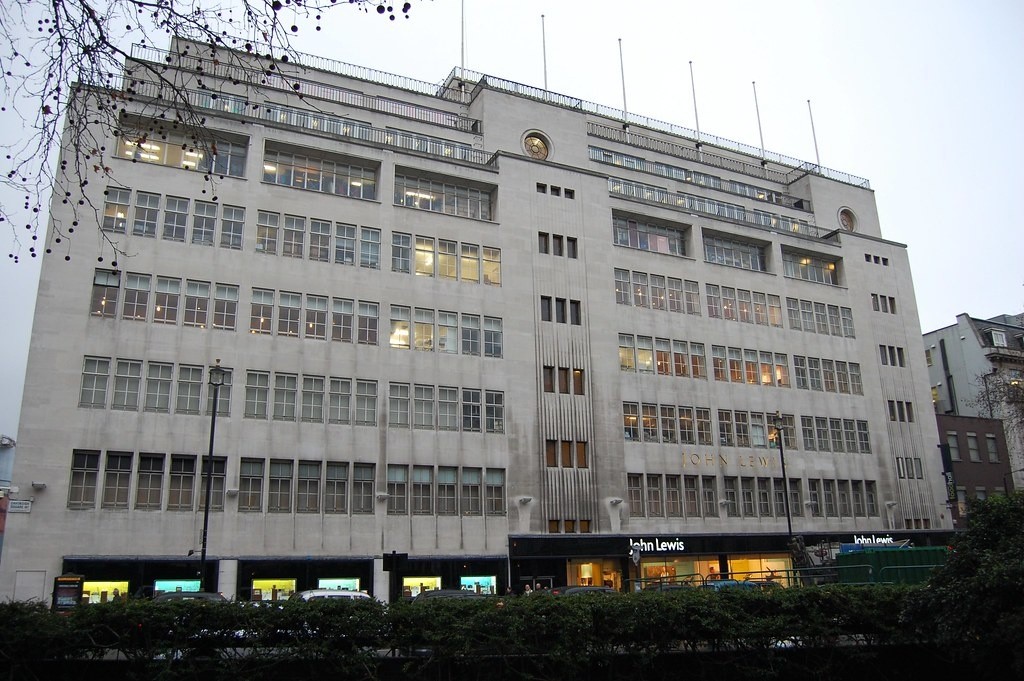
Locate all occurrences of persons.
[507,587,514,596]
[710,567,718,579]
[536,583,541,591]
[524,584,533,596]
[112,588,121,601]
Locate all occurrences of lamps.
[31,481,46,489]
[610,499,623,505]
[520,497,532,503]
[377,495,389,500]
[227,490,240,496]
[805,500,817,507]
[719,499,731,506]
[886,502,899,508]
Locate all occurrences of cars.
[150,567,762,604]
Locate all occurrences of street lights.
[772,411,800,587]
[199,357,225,592]
